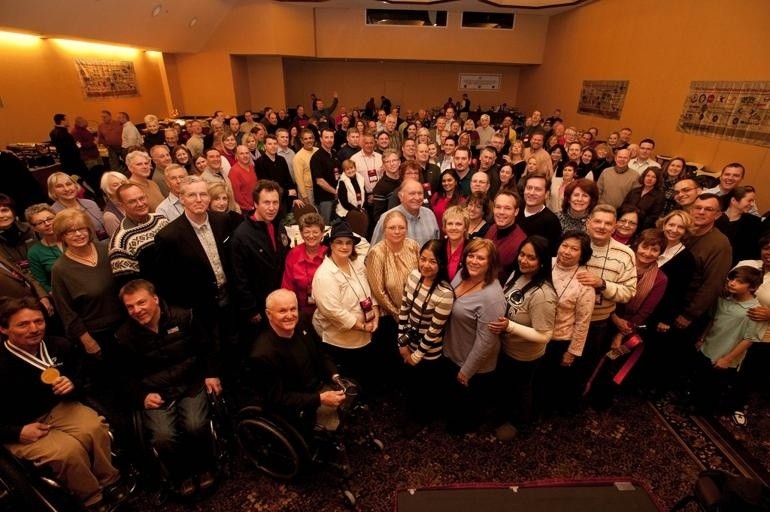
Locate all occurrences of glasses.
[30,215,54,228]
[62,225,88,235]
[673,186,697,196]
[618,219,638,226]
[690,204,719,211]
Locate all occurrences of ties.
[201,226,225,284]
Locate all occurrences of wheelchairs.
[131,387,228,497]
[230,376,385,509]
[0,396,137,512]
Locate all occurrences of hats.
[321,221,361,246]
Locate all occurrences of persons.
[1,94,769,512]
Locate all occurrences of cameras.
[397,327,423,348]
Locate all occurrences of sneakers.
[107,481,129,504]
[732,410,747,426]
[199,470,215,488]
[496,423,516,441]
[180,476,195,496]
[92,501,111,512]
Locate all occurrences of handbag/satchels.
[345,209,369,237]
